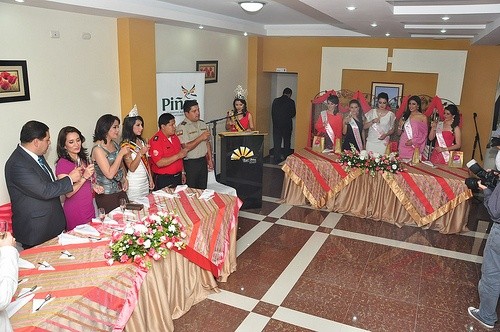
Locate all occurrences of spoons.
[61,252,73,257]
[38,263,50,268]
[18,285,37,298]
[36,294,51,311]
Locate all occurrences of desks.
[6,186,239,332]
[278,147,475,235]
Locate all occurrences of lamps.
[240,2,265,13]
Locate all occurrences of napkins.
[7,184,216,322]
[421,161,433,167]
[322,149,334,152]
[402,158,412,163]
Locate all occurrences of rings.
[14,238,15,240]
[409,144,410,145]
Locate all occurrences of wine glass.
[322,110,439,131]
[206,126,212,142]
[87,156,95,180]
[120,198,127,221]
[123,139,130,159]
[183,142,188,159]
[98,208,105,234]
[144,138,150,157]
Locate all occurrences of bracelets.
[398,127,401,130]
[82,176,88,180]
[345,122,347,125]
[138,152,142,157]
[181,172,186,175]
[386,133,389,136]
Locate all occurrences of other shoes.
[468,306,494,328]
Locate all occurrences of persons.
[90,114,131,215]
[148,113,188,191]
[468,168,500,328]
[120,104,155,201]
[55,126,104,232]
[495,135,500,171]
[429,104,461,166]
[4,120,83,251]
[226,85,255,131]
[271,88,296,163]
[342,99,366,153]
[0,232,18,332]
[363,92,396,153]
[175,100,213,190]
[315,95,342,150]
[396,95,428,159]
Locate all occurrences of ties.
[39,158,53,182]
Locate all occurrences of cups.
[74,158,82,167]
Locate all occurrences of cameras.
[465,159,498,193]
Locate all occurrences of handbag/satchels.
[126,203,144,210]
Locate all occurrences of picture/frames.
[0,59,30,103]
[196,59,219,83]
[370,82,403,110]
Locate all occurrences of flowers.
[102,211,188,267]
[341,150,399,171]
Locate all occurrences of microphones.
[241,109,246,115]
[473,113,477,119]
